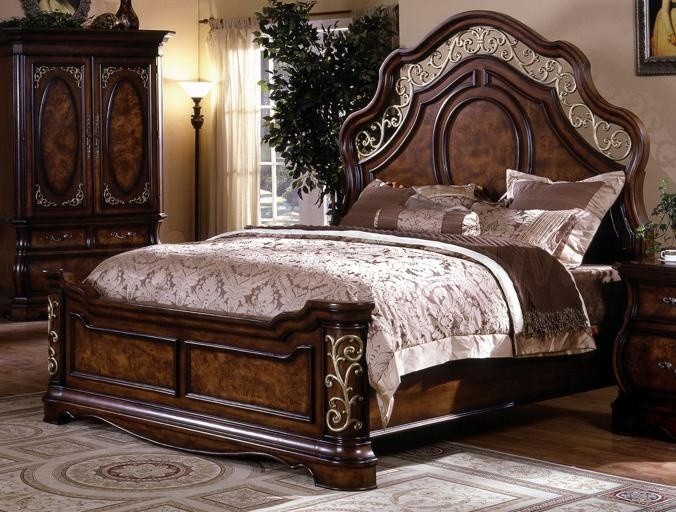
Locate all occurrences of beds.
[41,10,654,492]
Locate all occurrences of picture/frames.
[635,0,676,78]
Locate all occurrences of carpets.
[0,389,676,512]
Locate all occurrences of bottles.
[116,0,140,30]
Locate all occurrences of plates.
[658,258,676,265]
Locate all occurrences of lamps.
[179,81,214,241]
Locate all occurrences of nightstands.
[607,254,676,444]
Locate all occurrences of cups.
[630,233,646,264]
[661,250,676,260]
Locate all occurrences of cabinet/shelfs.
[0,0,176,319]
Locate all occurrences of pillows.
[330,168,625,268]
